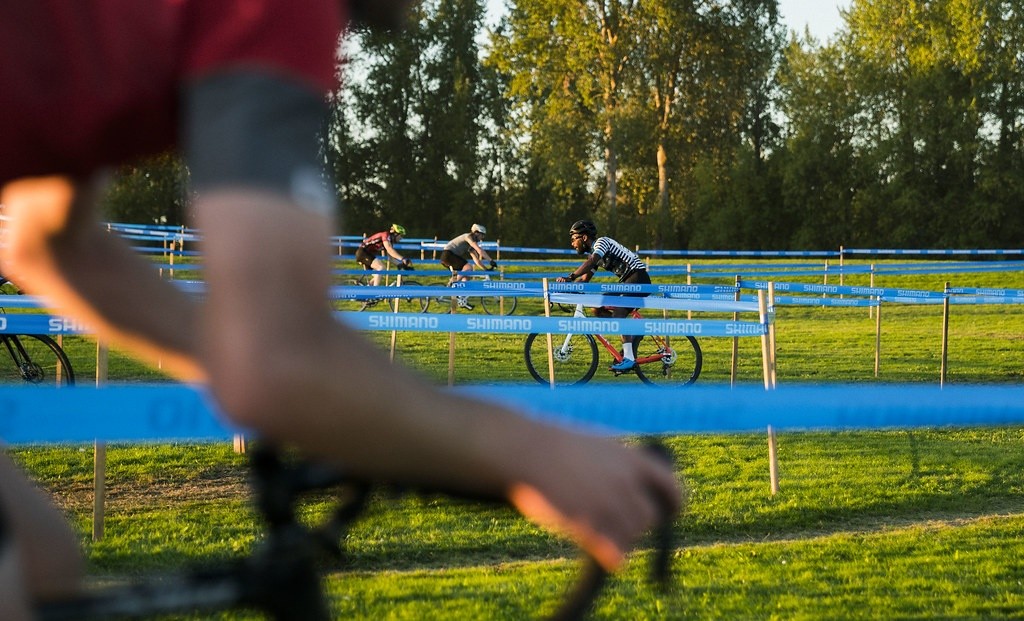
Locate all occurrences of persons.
[350,224,412,301]
[0,0,680,621]
[441,223,497,311]
[555,219,652,371]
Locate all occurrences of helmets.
[390,224,405,236]
[569,220,597,237]
[471,223,486,234]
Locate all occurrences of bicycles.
[0,278,75,387]
[524,278,704,391]
[420,264,518,318]
[328,260,430,314]
[0,436,679,621]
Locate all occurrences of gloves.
[490,261,497,267]
[402,257,409,265]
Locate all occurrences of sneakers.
[611,357,637,370]
